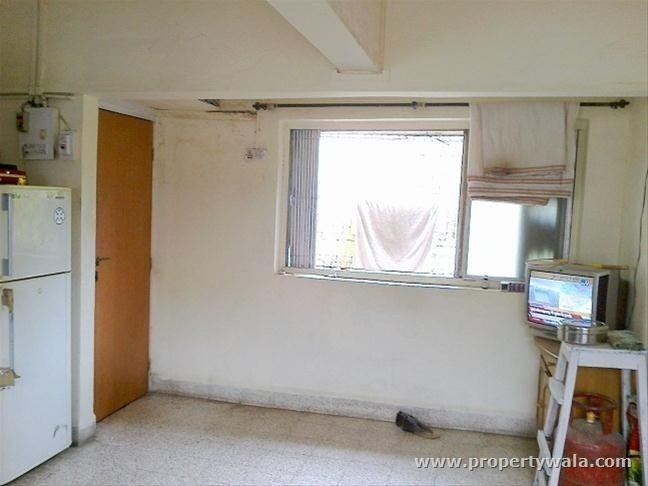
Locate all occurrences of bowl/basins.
[556,317,610,345]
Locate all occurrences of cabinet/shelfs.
[532,337,636,447]
[531,341,648,486]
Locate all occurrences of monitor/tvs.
[523,257,634,344]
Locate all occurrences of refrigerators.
[0,184,73,485]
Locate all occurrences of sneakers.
[397,411,440,439]
